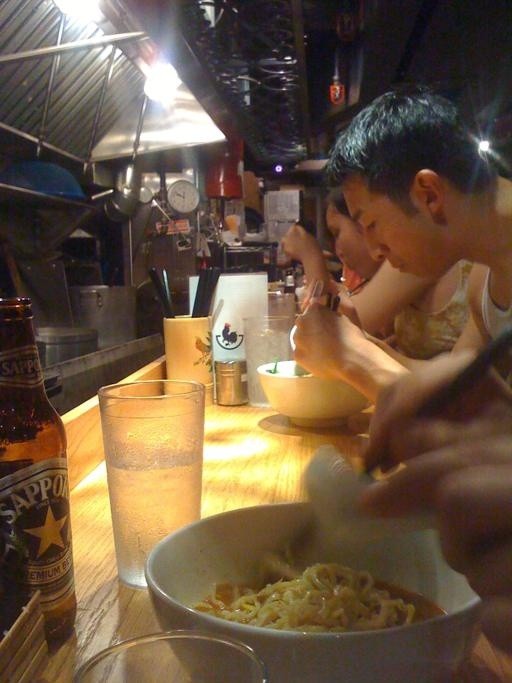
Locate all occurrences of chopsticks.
[146,261,220,318]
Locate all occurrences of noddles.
[189,563,417,634]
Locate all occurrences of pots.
[71,284,139,348]
[33,326,99,368]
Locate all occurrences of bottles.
[1,294,76,650]
[284,268,302,311]
[215,359,249,407]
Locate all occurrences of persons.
[281,183,476,366]
[360,344,511,658]
[286,81,511,405]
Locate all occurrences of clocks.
[167,180,200,213]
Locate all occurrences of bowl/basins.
[255,358,375,427]
[142,496,491,682]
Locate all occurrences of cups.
[242,312,292,407]
[271,293,299,324]
[69,627,275,681]
[96,377,208,593]
[162,313,218,405]
